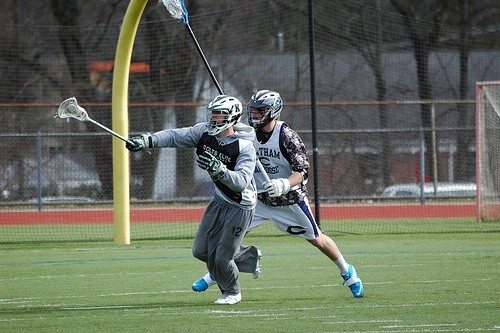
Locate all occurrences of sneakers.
[214,292,242,305]
[192,273,218,292]
[251,245,262,279]
[340,264,364,298]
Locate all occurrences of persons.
[191,89,364,298]
[126,95,263,304]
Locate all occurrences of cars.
[0,147,105,203]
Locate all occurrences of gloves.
[196,151,227,182]
[125,133,155,152]
[261,178,290,197]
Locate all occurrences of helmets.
[204,95,243,136]
[247,89,283,128]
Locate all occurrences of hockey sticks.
[160,0,226,95]
[55,96,139,146]
